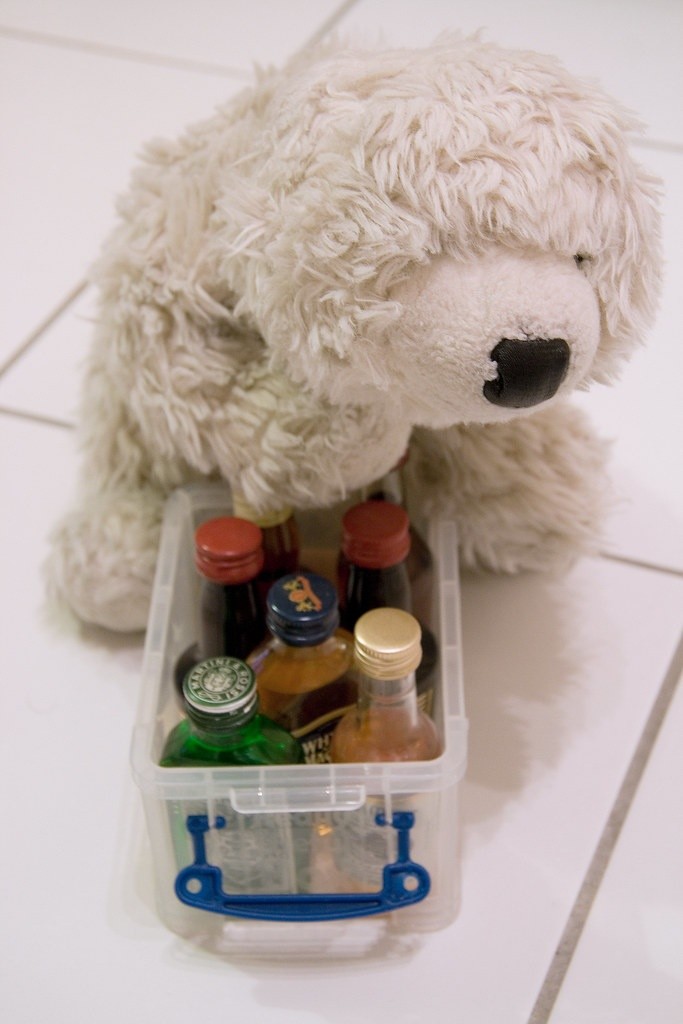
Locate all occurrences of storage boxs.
[128,479,469,956]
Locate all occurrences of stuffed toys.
[41,28,662,640]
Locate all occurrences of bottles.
[327,605,445,920]
[334,445,439,649]
[335,500,437,737]
[238,569,360,837]
[159,655,311,912]
[227,481,312,590]
[176,517,282,705]
